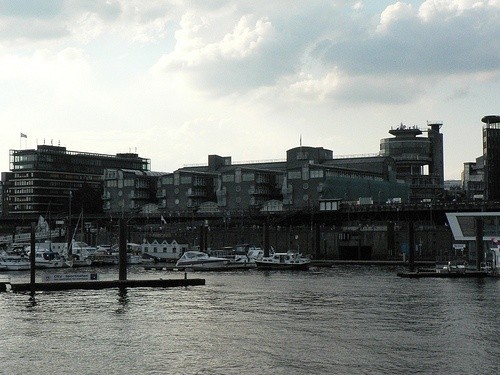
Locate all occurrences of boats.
[256,252,311,270]
[0,216,274,272]
